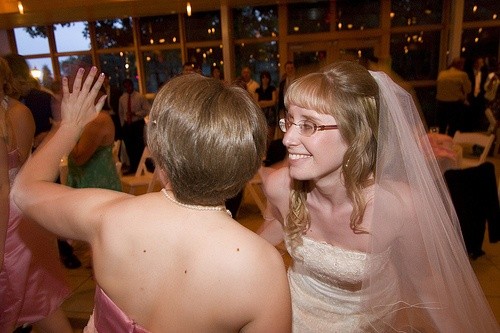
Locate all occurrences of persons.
[0,52,500,269]
[1,60,70,333]
[260,59,500,333]
[13,63,293,333]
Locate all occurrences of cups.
[430,127,439,136]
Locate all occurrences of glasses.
[278,117,339,137]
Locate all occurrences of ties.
[128,94,132,127]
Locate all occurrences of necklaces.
[161,188,233,218]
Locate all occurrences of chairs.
[58,130,496,258]
